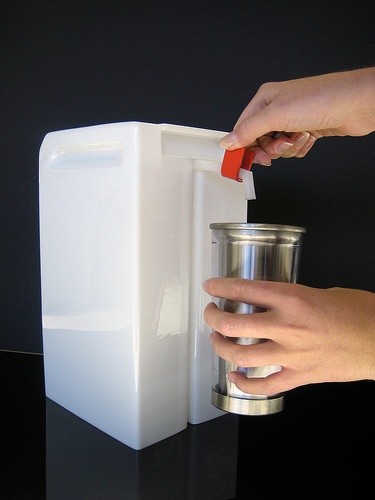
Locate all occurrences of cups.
[208,223,307,420]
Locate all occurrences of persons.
[204,65,375,396]
